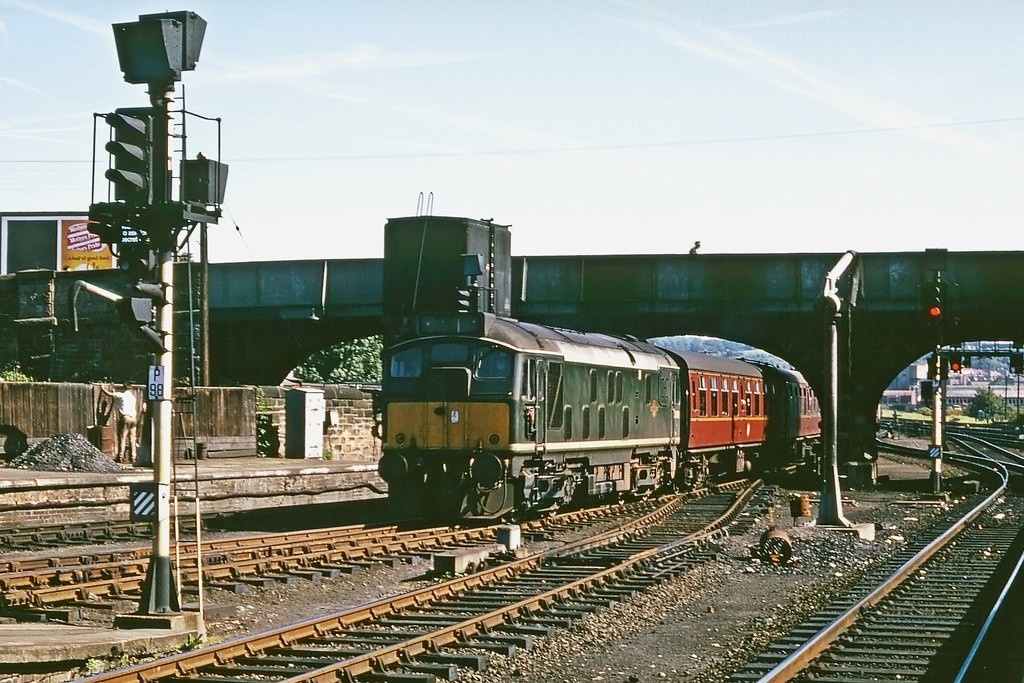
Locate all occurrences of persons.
[689,241,701,254]
[101,380,137,463]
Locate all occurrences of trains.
[371,312,826,528]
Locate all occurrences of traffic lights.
[950,352,962,372]
[454,284,480,316]
[114,295,154,331]
[104,107,154,206]
[928,280,944,324]
[964,354,971,368]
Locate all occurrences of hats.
[695,241,700,245]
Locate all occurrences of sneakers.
[130,458,137,464]
[113,457,122,463]
